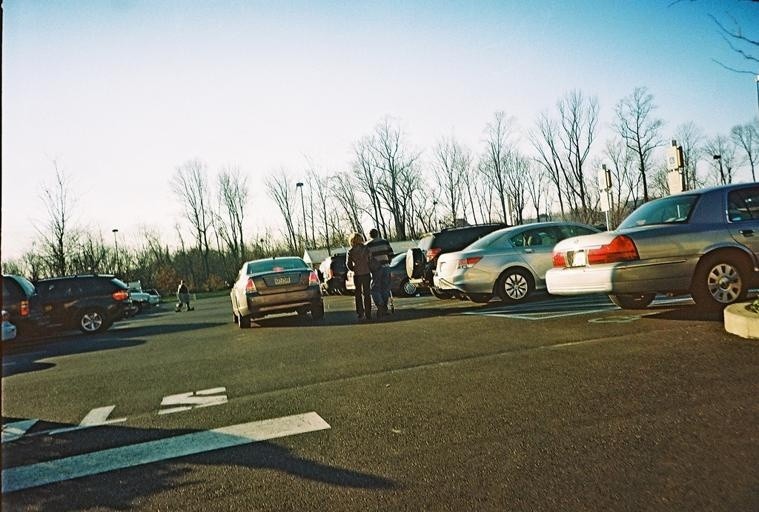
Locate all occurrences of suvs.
[315,253,347,295]
[406,223,513,301]
[33,274,133,335]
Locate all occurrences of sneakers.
[357,307,391,321]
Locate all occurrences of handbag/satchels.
[367,249,381,272]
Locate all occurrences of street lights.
[297,183,307,245]
[113,229,120,274]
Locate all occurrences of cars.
[0,274,48,355]
[545,182,759,312]
[433,220,602,306]
[128,285,161,315]
[229,256,324,328]
[371,251,419,298]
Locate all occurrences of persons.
[363,228,395,317]
[175,280,194,313]
[345,232,372,320]
